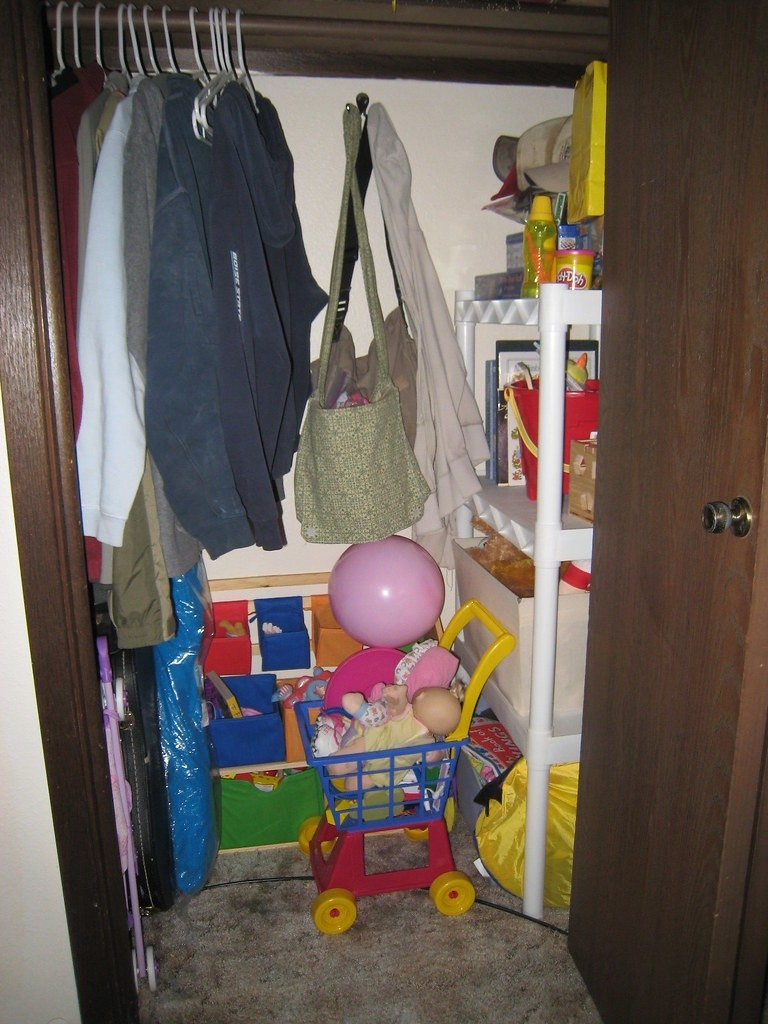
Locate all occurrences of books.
[206,670,243,718]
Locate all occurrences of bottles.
[520,195,555,299]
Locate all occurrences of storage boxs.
[204,600,252,676]
[450,537,588,717]
[311,591,363,667]
[569,439,596,521]
[214,767,325,851]
[255,595,311,671]
[474,272,523,300]
[506,225,576,272]
[566,61,607,225]
[457,749,490,833]
[278,678,323,763]
[208,674,286,769]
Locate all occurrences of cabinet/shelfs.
[453,283,603,919]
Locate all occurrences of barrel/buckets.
[505,374,602,501]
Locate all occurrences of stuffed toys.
[312,638,460,766]
[328,678,464,791]
[465,513,573,600]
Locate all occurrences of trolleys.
[299,599,516,934]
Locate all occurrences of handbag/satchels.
[294,378,432,545]
[308,306,419,446]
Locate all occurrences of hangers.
[51,0,257,144]
